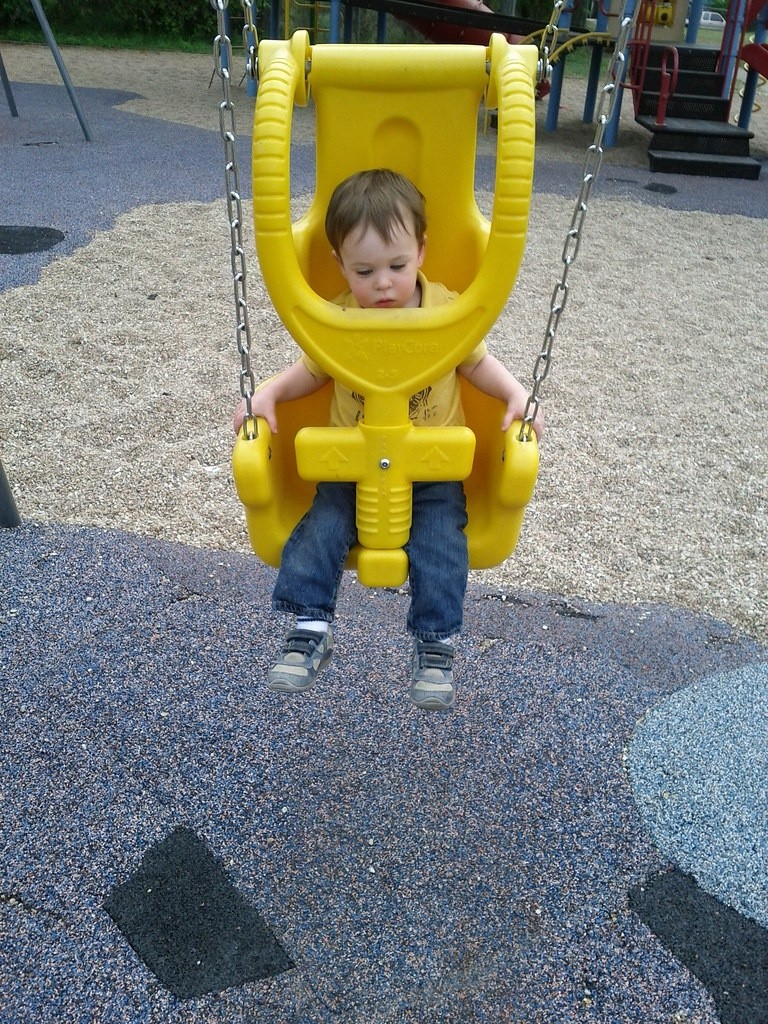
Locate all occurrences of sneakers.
[267,626,333,692]
[409,639,455,710]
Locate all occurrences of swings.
[207,0,639,591]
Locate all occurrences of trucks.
[685,11,726,29]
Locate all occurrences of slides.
[740,42,768,81]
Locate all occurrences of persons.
[231,168,545,707]
